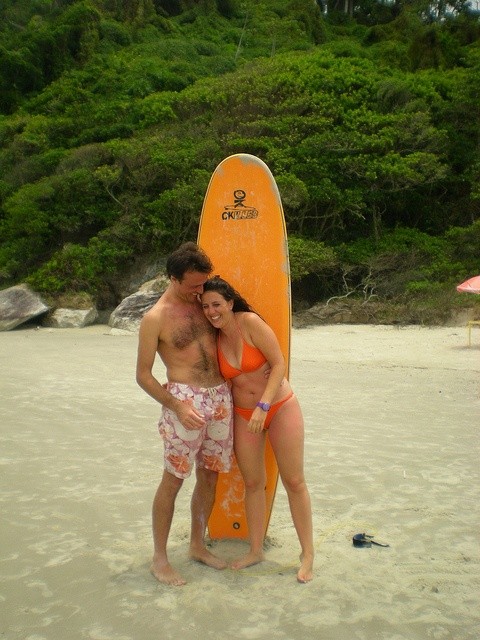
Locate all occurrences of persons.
[136,241,234,586]
[199,274,315,584]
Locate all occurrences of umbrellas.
[456,276,480,295]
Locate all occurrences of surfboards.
[196,153,291,540]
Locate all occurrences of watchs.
[256,402,271,411]
